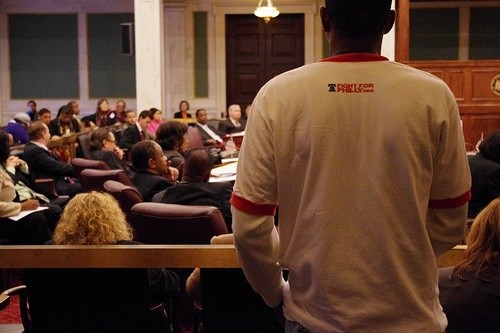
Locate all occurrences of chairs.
[35,158,229,333]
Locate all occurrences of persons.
[6,101,86,159]
[3,156,71,222]
[230,0,471,332]
[26,190,180,333]
[468,132,500,219]
[88,128,135,182]
[153,148,233,236]
[0,129,51,246]
[437,200,500,333]
[23,123,84,195]
[195,109,229,147]
[157,121,190,182]
[219,104,247,134]
[87,98,164,149]
[131,140,177,201]
[174,100,192,119]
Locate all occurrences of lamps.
[254,0,280,24]
